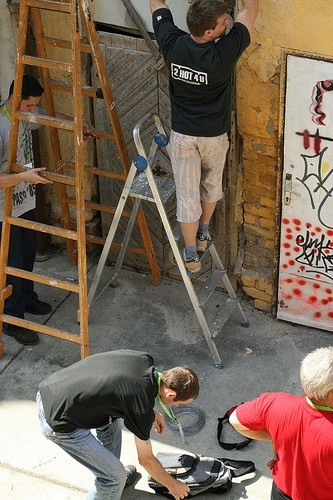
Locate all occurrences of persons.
[229,346,333,500]
[35,348,200,500]
[0,76,53,347]
[149,0,261,272]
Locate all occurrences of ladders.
[77,112,250,369]
[0,0,163,366]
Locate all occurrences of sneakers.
[2,322,39,346]
[169,247,201,272]
[24,299,51,315]
[196,238,208,251]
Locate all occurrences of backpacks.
[148,451,256,498]
